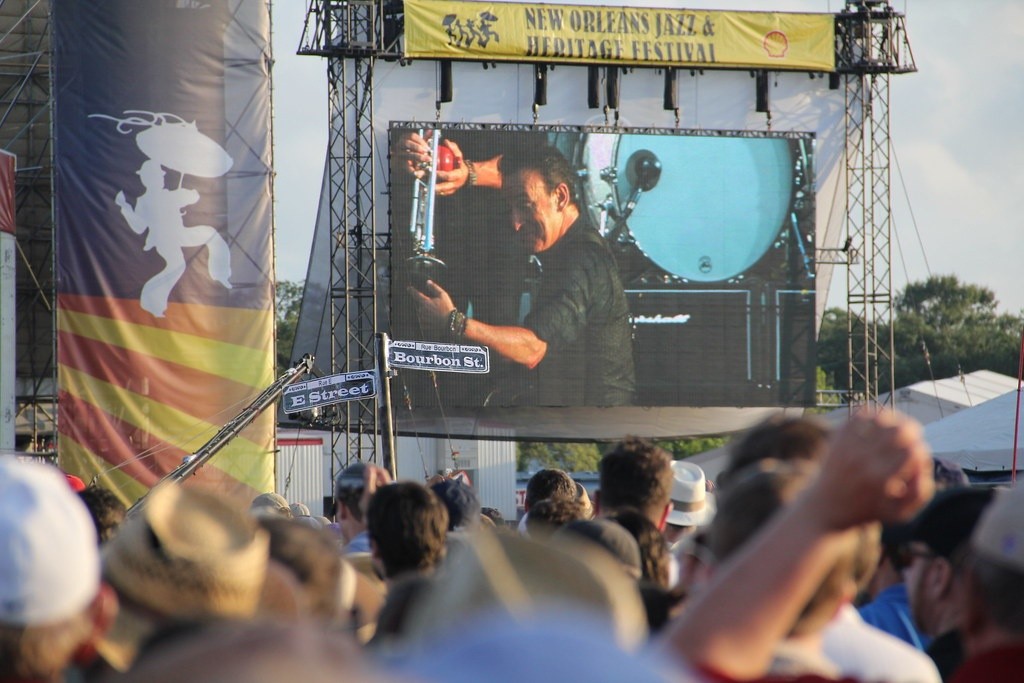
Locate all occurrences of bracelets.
[463,158,478,191]
[449,309,466,338]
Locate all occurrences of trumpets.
[403,129,451,289]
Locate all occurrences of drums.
[571,133,793,285]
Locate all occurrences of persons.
[395,127,547,328]
[406,142,642,407]
[0,399,1024,683]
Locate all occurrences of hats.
[103,479,271,630]
[397,517,655,656]
[665,459,718,528]
[0,452,101,628]
[970,479,1024,569]
